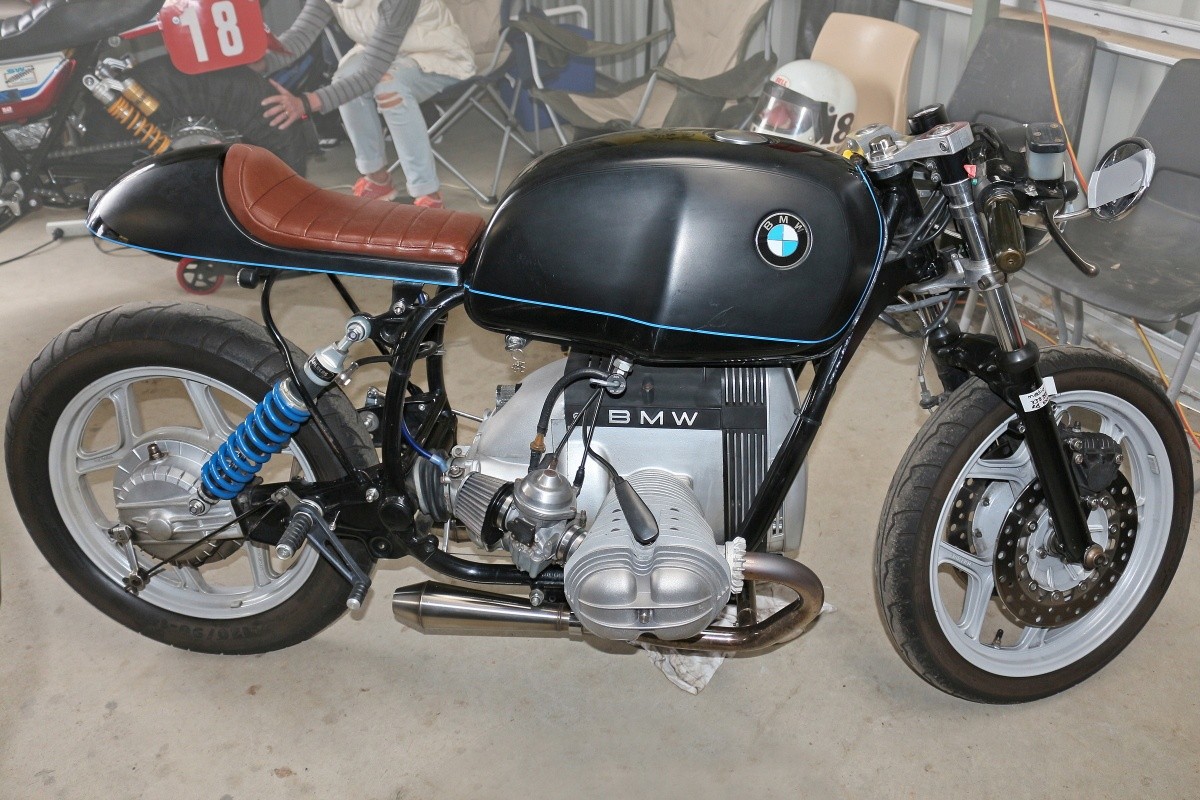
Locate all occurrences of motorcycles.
[0,0,308,233]
[6,105,1194,704]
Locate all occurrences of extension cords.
[46,219,92,238]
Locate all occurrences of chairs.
[876,16,1099,347]
[971,59,1200,495]
[325,0,587,205]
[796,0,901,73]
[811,12,921,162]
[508,0,778,142]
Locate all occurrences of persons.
[249,0,478,208]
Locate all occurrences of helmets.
[749,58,855,154]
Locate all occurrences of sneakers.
[413,193,443,208]
[350,174,397,201]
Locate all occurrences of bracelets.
[296,92,312,114]
